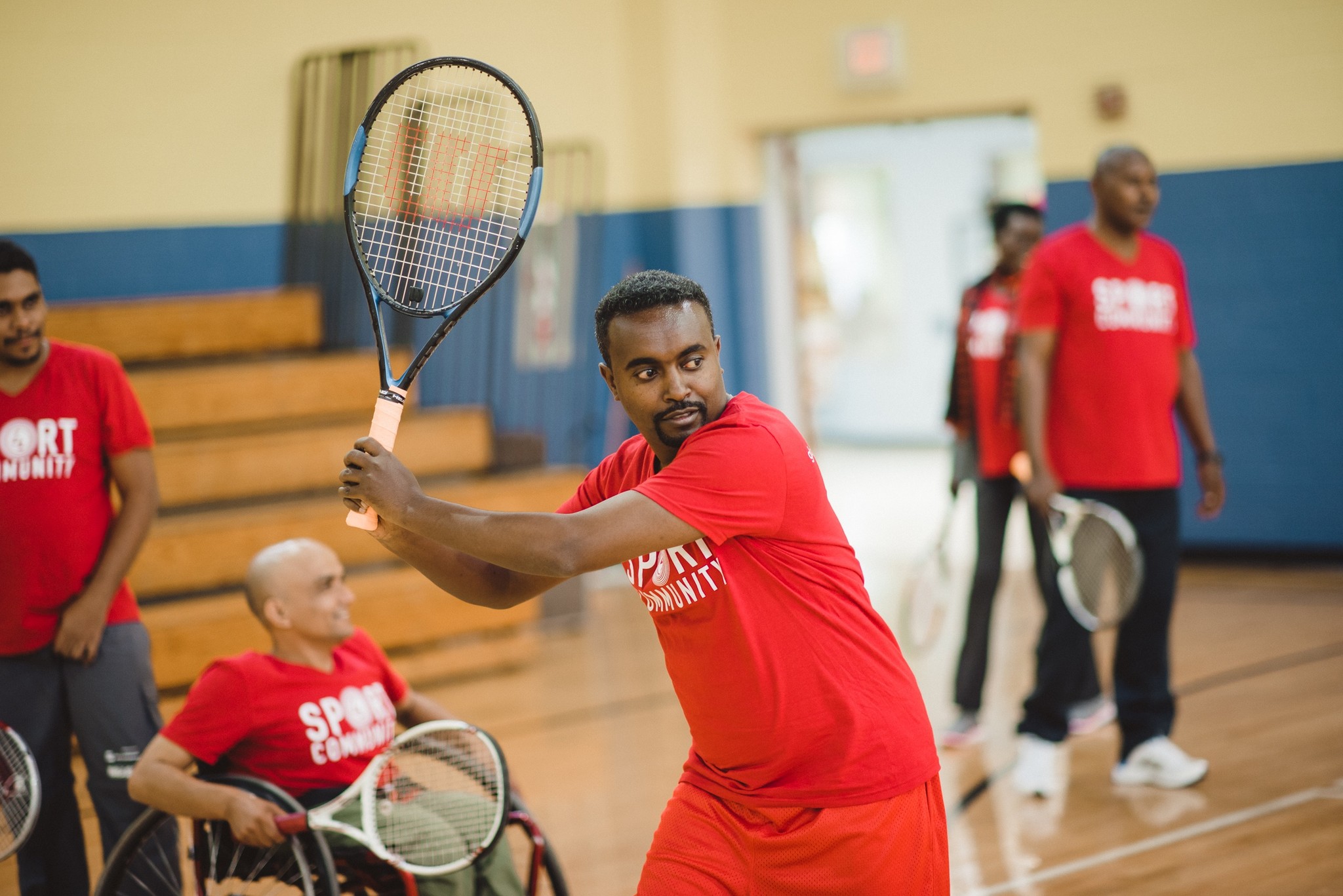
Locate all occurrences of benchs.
[34,282,589,715]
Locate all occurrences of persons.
[940,200,1117,755]
[0,241,182,896]
[1003,143,1228,798]
[129,538,528,896]
[340,267,951,896]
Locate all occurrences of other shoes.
[1012,733,1065,797]
[1068,690,1118,737]
[938,702,979,750]
[1110,733,1211,788]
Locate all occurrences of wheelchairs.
[93,716,567,896]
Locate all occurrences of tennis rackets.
[1009,447,1143,631]
[272,717,507,878]
[345,54,544,531]
[901,474,965,658]
[0,721,40,860]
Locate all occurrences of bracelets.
[1195,448,1224,467]
